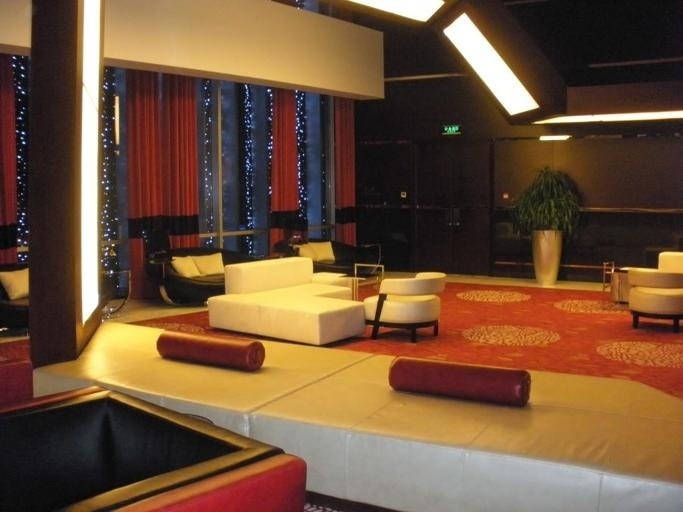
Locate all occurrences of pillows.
[0,267,28,299]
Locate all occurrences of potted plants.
[506,165,583,289]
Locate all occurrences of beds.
[152,236,379,300]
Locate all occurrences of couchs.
[207,255,365,344]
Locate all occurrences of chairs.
[364,269,446,341]
[627,251,681,329]
